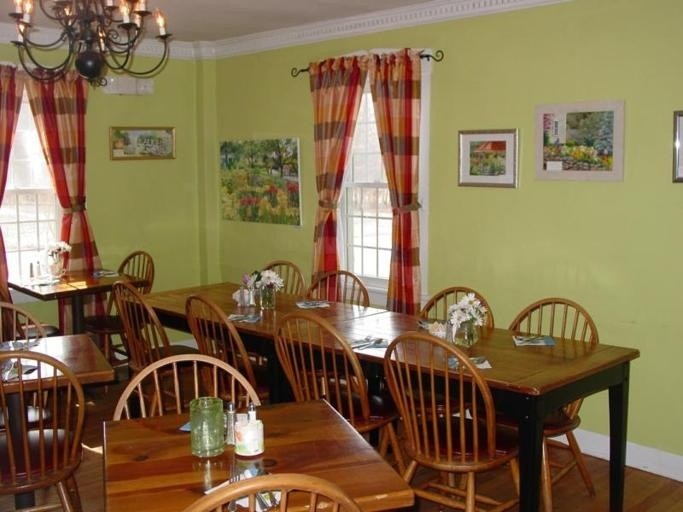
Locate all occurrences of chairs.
[479,297,598,512]
[0,350,84,512]
[273,311,406,477]
[8,291,60,338]
[82,249,155,365]
[0,301,46,429]
[421,284,495,329]
[185,295,271,410]
[112,280,208,417]
[114,354,262,421]
[384,331,520,512]
[308,271,370,308]
[262,258,305,299]
[184,472,365,512]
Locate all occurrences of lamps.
[0,0,173,88]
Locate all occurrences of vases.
[51,259,66,280]
[456,322,479,349]
[258,288,276,310]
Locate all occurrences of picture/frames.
[458,126,520,188]
[217,137,306,226]
[533,100,627,178]
[109,125,175,160]
[673,109,682,184]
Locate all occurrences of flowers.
[242,268,285,311]
[47,240,73,280]
[444,291,487,347]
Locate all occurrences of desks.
[0,335,114,433]
[101,398,414,512]
[124,281,640,511]
[9,268,152,337]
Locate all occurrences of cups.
[188,396,231,458]
[238,287,262,324]
